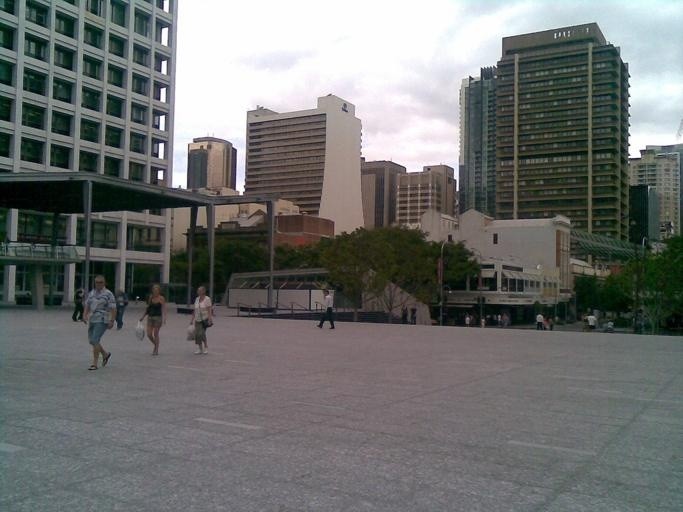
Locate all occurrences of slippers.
[103,351,111,366]
[88,365,97,370]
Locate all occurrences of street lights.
[641,237,650,334]
[470,248,482,328]
[438,240,454,326]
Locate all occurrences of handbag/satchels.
[202,319,213,328]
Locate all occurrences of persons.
[139,284,166,355]
[316,290,335,329]
[583,313,597,330]
[537,314,554,329]
[115,288,128,330]
[604,319,614,333]
[72,288,84,322]
[464,311,510,328]
[190,286,212,354]
[83,275,117,369]
